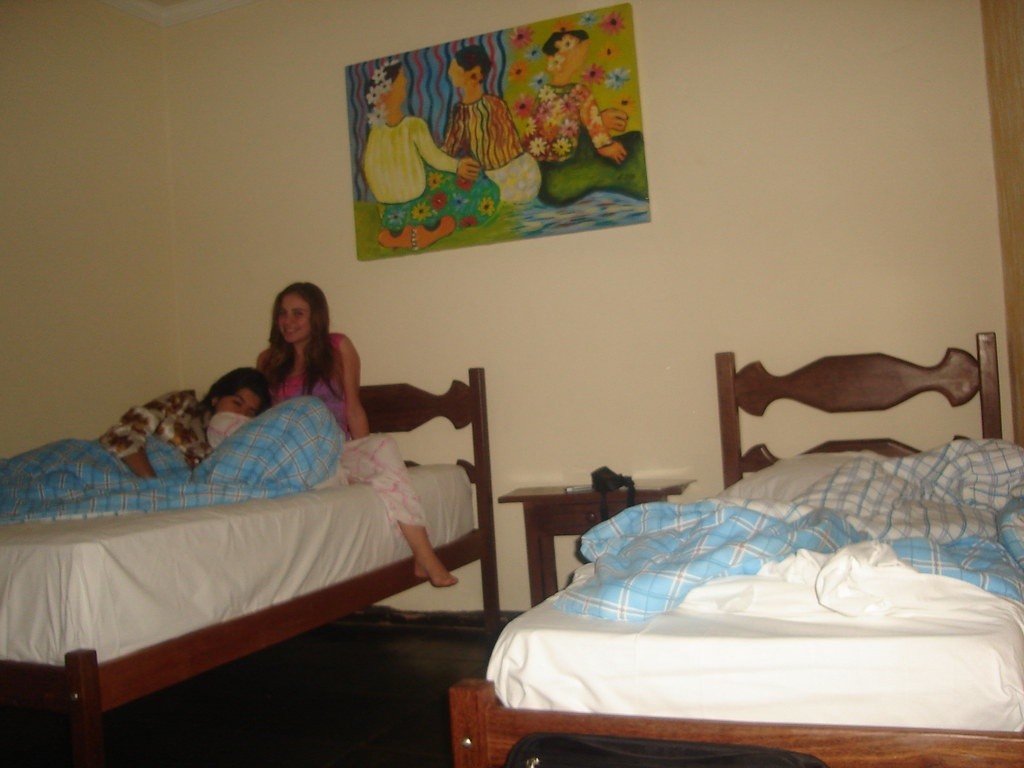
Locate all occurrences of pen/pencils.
[564,485,592,491]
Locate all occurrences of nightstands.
[498,479,696,610]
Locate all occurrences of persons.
[99,368,272,476]
[254,282,460,588]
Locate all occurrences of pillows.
[711,450,862,501]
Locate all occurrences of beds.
[0,366,497,768]
[447,332,1024,768]
